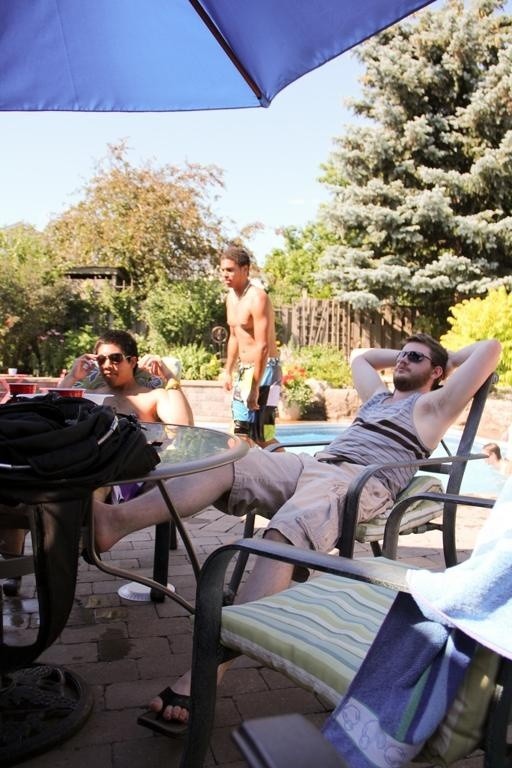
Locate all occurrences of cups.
[9,383,38,404]
[48,389,87,398]
[9,368,18,377]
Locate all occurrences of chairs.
[223,372,500,607]
[0,354,182,604]
[178,491,511,768]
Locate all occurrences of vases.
[279,396,301,421]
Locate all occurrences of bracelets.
[161,377,180,391]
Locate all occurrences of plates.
[118,581,176,602]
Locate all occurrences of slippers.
[137,685,191,739]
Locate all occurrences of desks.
[1,421,251,767]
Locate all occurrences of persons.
[217,244,287,454]
[56,329,196,505]
[480,442,512,476]
[88,330,503,739]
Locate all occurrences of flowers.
[276,367,311,407]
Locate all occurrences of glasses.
[96,350,128,365]
[397,350,436,369]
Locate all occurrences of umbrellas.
[2,0,446,116]
